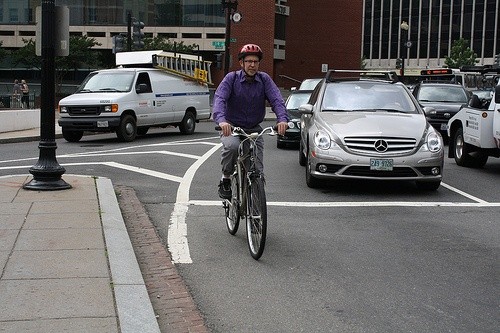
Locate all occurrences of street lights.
[399,19,409,83]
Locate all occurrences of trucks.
[449,79,500,167]
[58,67,211,143]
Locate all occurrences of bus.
[421,68,500,92]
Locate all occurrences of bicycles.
[214,124,290,259]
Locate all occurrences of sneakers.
[250,215,261,234]
[218,178,232,198]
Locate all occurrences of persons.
[12,80,22,108]
[20,80,30,109]
[212,43,288,234]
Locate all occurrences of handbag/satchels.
[23,84,28,93]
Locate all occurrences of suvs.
[298,69,445,192]
[412,75,469,143]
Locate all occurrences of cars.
[270,92,314,149]
[299,79,323,95]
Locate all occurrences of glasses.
[244,60,259,63]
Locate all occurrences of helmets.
[238,44,263,59]
